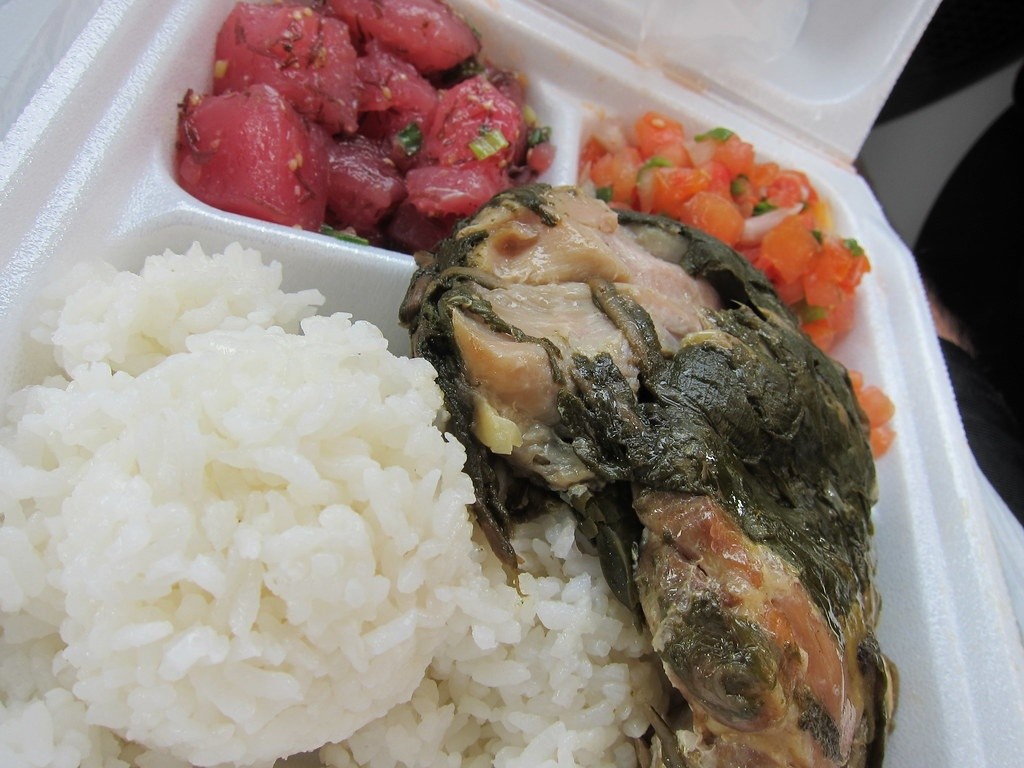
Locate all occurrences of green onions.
[395,102,865,322]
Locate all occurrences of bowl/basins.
[0,0,1024,768]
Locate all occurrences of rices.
[0,239,670,768]
